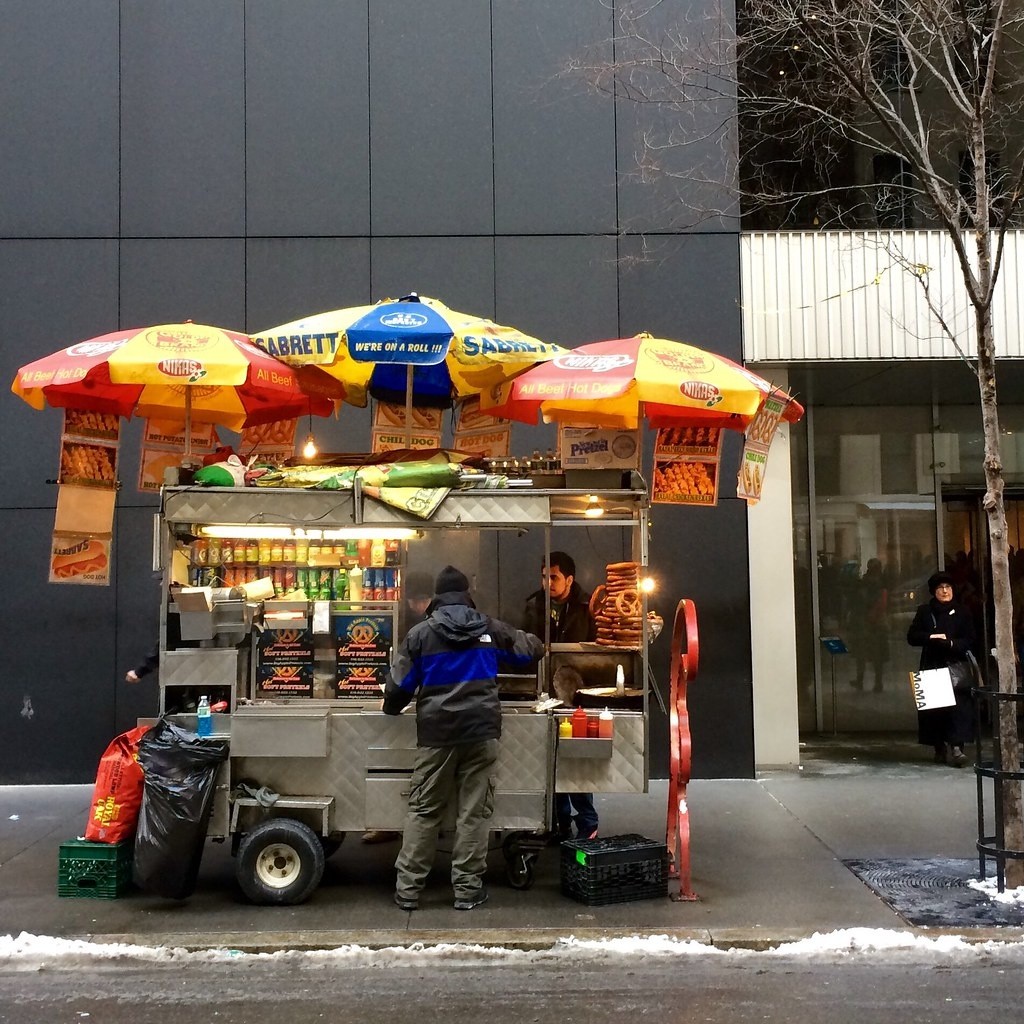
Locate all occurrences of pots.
[575,682,652,709]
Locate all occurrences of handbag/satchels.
[908,651,986,710]
[869,589,890,632]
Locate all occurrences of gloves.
[930,638,952,651]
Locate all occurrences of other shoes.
[850,679,883,693]
[362,831,396,842]
[933,746,967,767]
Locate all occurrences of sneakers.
[394,891,419,910]
[455,891,489,909]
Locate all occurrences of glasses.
[936,586,951,590]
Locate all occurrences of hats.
[435,566,468,593]
[405,571,434,599]
[929,573,956,595]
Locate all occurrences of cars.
[891,565,936,612]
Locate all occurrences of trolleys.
[157,481,663,908]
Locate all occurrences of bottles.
[197,696,212,737]
[588,717,599,738]
[559,717,572,737]
[189,539,398,567]
[570,705,587,738]
[599,706,613,738]
[349,563,362,610]
[334,569,349,610]
[488,447,563,474]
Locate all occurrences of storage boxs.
[56,834,133,902]
[561,831,673,907]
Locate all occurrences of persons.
[816,548,1024,695]
[125,572,197,716]
[907,571,970,765]
[381,565,545,909]
[402,571,435,639]
[519,550,598,843]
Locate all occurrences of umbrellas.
[12,320,345,460]
[250,292,569,447]
[481,332,806,480]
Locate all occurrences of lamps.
[585,496,604,519]
[190,522,425,542]
